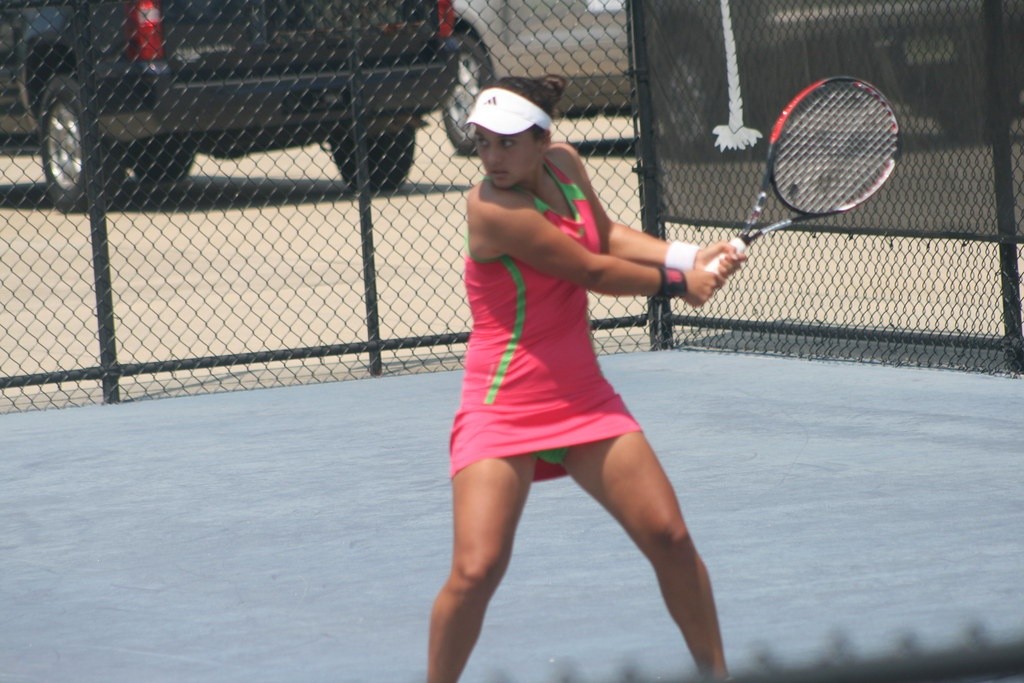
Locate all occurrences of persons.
[425,72,747,682]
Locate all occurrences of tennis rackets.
[701,74,902,275]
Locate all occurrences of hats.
[464,86,552,135]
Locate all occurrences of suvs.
[0,1,462,213]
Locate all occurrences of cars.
[442,2,1023,159]
